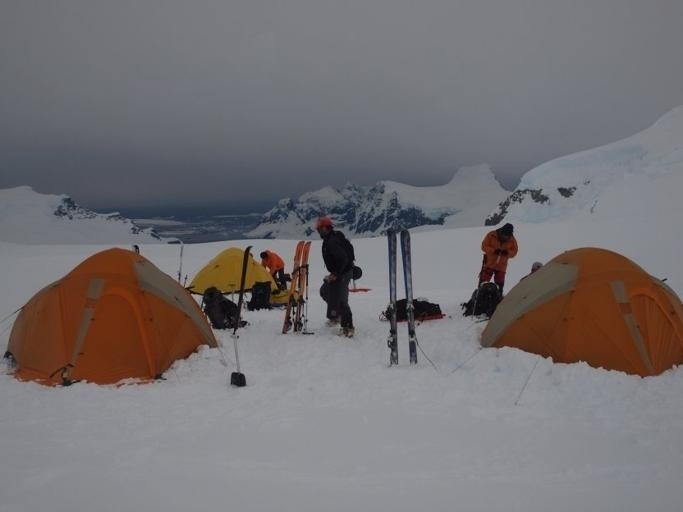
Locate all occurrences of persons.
[316,218,354,338]
[532,262,542,273]
[260,250,287,290]
[478,222,518,296]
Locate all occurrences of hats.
[532,262,541,269]
[260,252,266,258]
[503,224,513,233]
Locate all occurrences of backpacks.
[201,286,246,329]
[247,281,271,311]
[461,283,501,317]
[379,299,440,322]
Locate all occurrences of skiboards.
[233,246,252,333]
[178,240,184,284]
[381,228,417,366]
[282,241,311,334]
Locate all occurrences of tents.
[188,248,278,295]
[481,247,683,378]
[4,248,218,386]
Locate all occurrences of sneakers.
[339,327,354,338]
[325,318,341,327]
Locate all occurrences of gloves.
[501,250,507,256]
[495,248,500,255]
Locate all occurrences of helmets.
[318,217,333,226]
